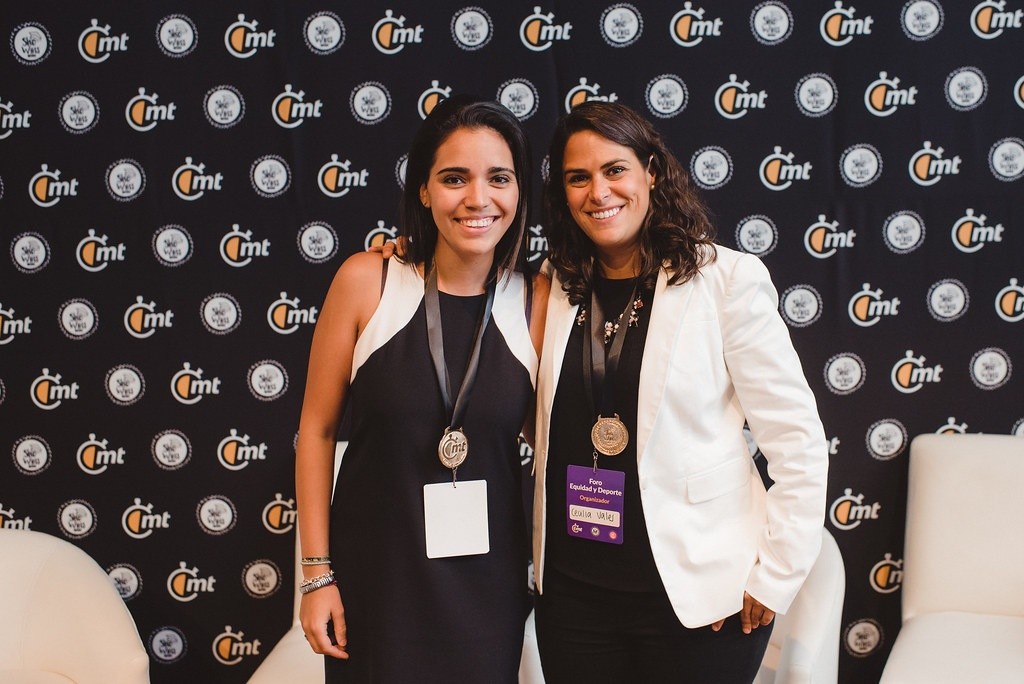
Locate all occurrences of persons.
[294,90,552,684]
[365,100,829,684]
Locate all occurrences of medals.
[438,425,468,469]
[591,412,629,456]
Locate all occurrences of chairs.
[246,442,348,684]
[0,527,151,684]
[518,527,846,684]
[879,434,1024,684]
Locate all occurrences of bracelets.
[298,570,335,588]
[299,575,335,594]
[301,556,331,565]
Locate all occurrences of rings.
[304,634,308,641]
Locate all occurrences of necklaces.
[576,292,643,345]
